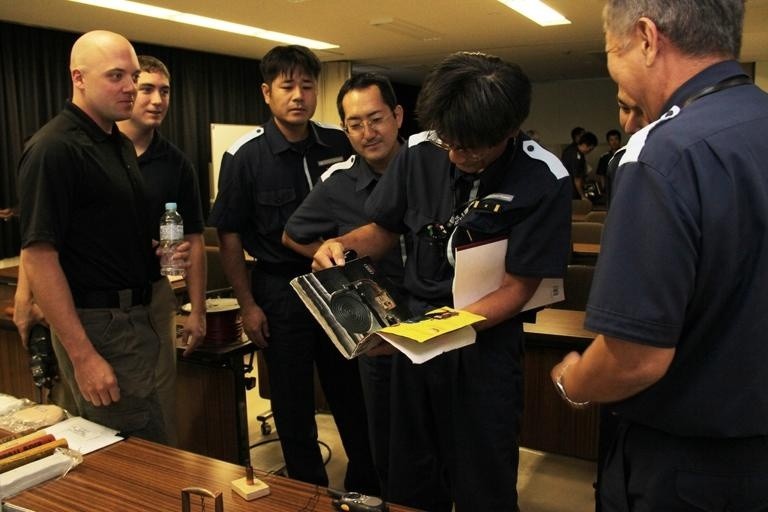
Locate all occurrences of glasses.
[343,114,391,137]
[427,128,491,162]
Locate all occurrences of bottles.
[157,200,191,281]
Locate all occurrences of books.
[289,254,487,365]
[452,235,566,334]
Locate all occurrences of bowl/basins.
[182,297,251,347]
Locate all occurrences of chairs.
[570,199,607,244]
[551,265,595,311]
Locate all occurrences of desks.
[520,306,600,463]
[0,431,416,510]
[0,256,250,466]
[571,242,601,265]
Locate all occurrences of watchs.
[555,366,594,410]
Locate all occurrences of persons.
[13,54,209,449]
[311,51,572,512]
[527,127,622,211]
[550,0,768,512]
[17,30,192,448]
[593,82,652,512]
[211,46,381,498]
[282,74,410,498]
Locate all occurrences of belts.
[73,290,153,310]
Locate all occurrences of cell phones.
[328,489,387,512]
